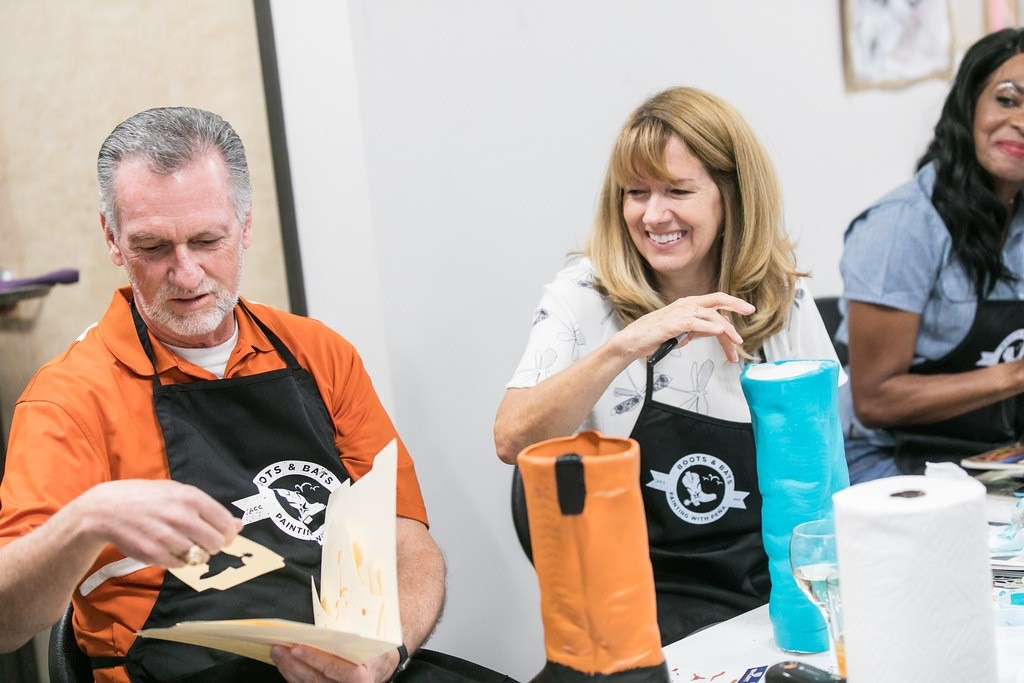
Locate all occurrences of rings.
[694,305,699,318]
[183,544,203,566]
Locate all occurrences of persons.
[493,87,909,648]
[835,30,1024,487]
[0,106,525,683]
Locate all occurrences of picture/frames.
[983,1,1020,36]
[840,0,959,93]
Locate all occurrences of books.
[960,442,1024,469]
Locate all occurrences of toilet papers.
[831,462,998,683]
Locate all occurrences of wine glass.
[790,520,847,680]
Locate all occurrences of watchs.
[389,644,410,683]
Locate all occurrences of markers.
[646,332,689,368]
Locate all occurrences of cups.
[986,481,1024,559]
[822,565,846,679]
